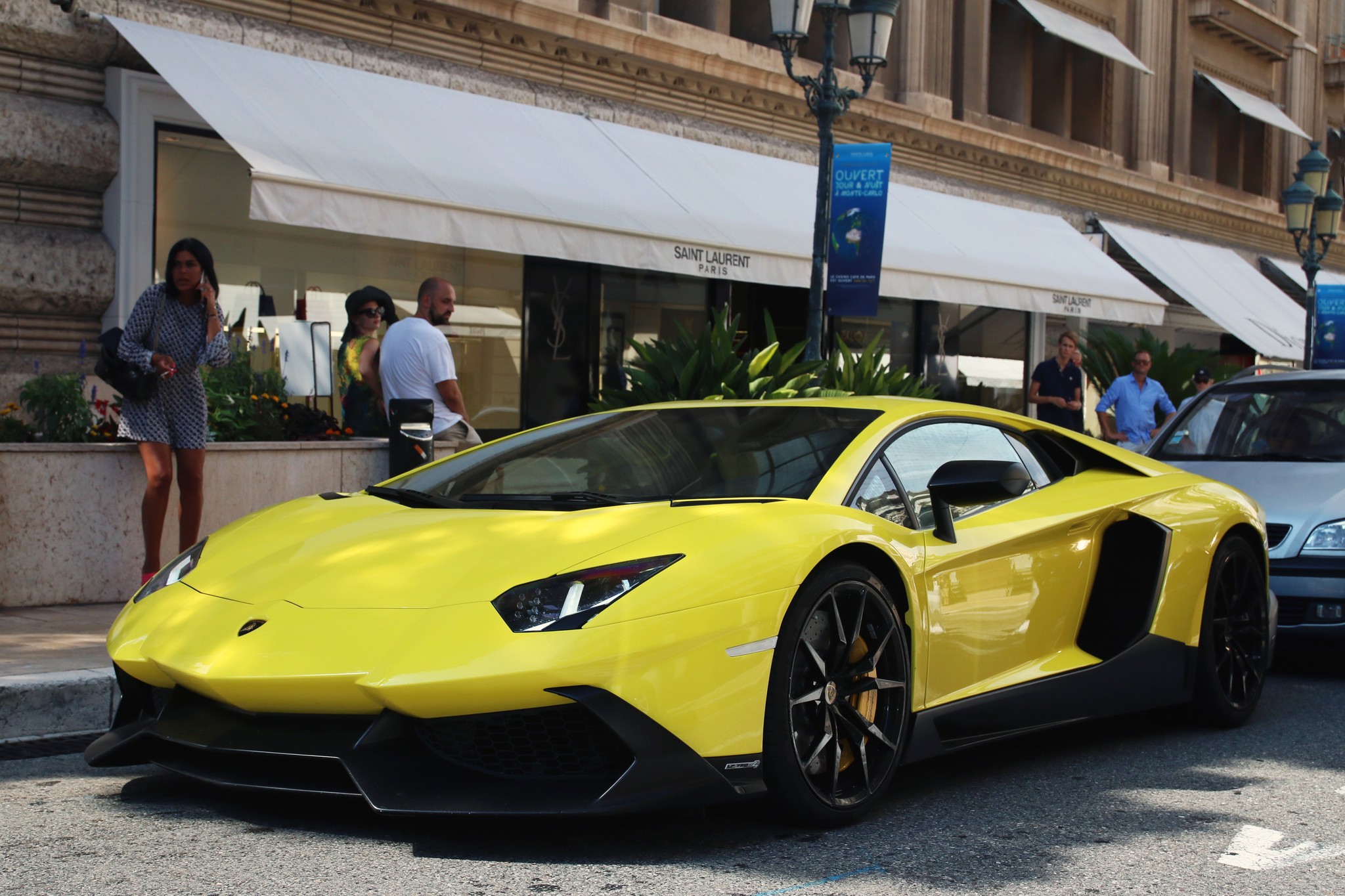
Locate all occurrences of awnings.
[1199,71,1316,144]
[95,14,1169,326]
[1016,0,1160,80]
[1086,216,1345,361]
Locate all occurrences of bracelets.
[1076,401,1082,411]
[207,297,218,309]
[207,313,218,317]
[1045,395,1049,405]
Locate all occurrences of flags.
[828,141,899,319]
[1310,284,1344,367]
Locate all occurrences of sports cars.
[84,393,1280,821]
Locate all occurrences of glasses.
[1072,358,1082,365]
[357,306,386,318]
[1134,359,1151,365]
[1194,377,1209,384]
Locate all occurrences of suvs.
[1136,363,1344,647]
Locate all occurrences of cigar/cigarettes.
[160,368,175,377]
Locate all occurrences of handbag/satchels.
[96,327,154,404]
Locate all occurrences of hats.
[1195,365,1213,378]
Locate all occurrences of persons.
[110,235,228,592]
[1027,330,1086,434]
[1070,349,1087,424]
[1171,368,1231,458]
[336,288,395,438]
[1094,351,1177,455]
[379,276,485,453]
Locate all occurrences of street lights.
[767,0,902,378]
[1283,141,1342,372]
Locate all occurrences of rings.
[209,288,214,291]
